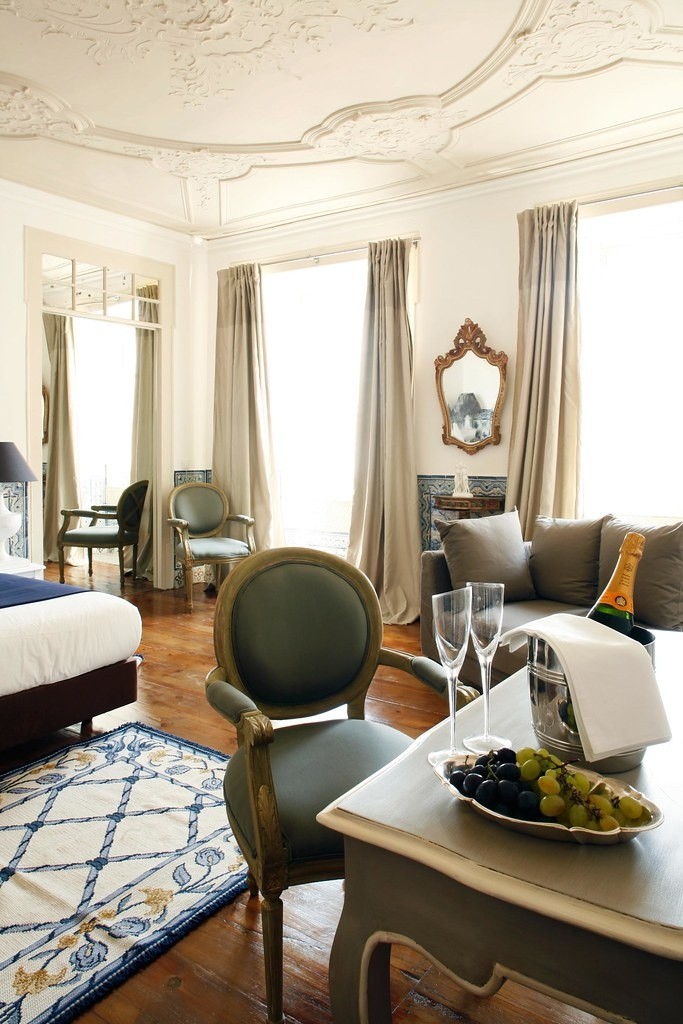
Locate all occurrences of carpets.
[0,722,249,1024]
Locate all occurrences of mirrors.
[434,318,509,455]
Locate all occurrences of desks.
[430,495,507,520]
[314,644,683,1024]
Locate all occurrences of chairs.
[204,544,481,1021]
[55,480,149,589]
[165,481,256,612]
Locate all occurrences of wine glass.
[430,586,474,763]
[463,581,512,756]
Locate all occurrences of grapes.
[558,700,576,731]
[448,748,644,832]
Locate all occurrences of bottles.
[585,531,645,638]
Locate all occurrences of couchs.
[416,540,645,694]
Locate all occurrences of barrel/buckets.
[524,620,656,773]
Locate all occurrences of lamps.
[0,441,39,561]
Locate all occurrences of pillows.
[433,505,537,611]
[597,513,683,632]
[526,514,616,607]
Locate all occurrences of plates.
[432,753,665,843]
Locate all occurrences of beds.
[0,571,143,753]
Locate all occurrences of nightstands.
[0,558,47,581]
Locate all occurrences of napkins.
[496,613,671,764]
